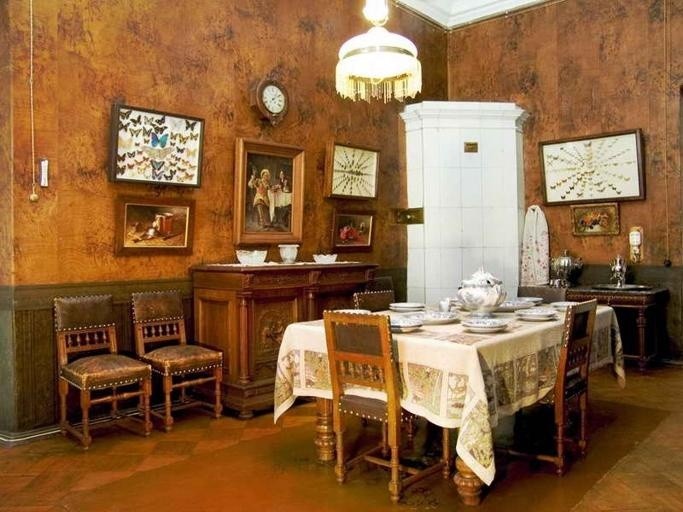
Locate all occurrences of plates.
[387,318,424,333]
[461,295,578,334]
[408,310,460,325]
[335,309,370,315]
[388,302,425,312]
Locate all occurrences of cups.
[438,302,451,312]
[154,212,173,234]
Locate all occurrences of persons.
[247,166,290,227]
[146,214,161,240]
[128,222,145,241]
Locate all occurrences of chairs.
[508,298,597,476]
[48,296,155,449]
[128,294,225,433]
[515,285,568,303]
[351,289,396,312]
[322,311,450,503]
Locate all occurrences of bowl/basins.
[234,249,267,265]
[311,253,339,264]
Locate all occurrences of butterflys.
[115,109,200,183]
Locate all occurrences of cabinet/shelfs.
[194,261,384,421]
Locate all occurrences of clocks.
[249,76,289,129]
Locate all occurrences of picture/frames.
[568,202,621,237]
[538,127,646,207]
[331,207,376,248]
[324,136,381,202]
[110,105,203,184]
[110,197,195,257]
[230,137,305,245]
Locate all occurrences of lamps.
[334,1,423,105]
